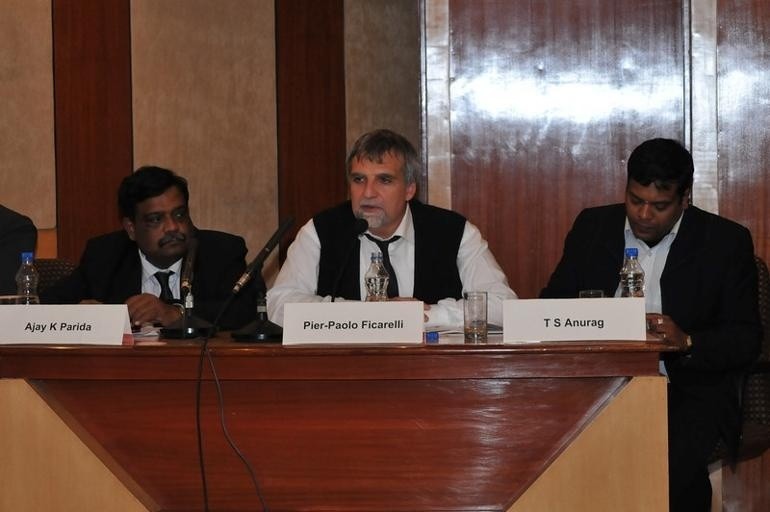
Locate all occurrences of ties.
[153,271,176,305]
[364,232,401,299]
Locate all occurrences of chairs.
[34,258,75,295]
[713,255,770,462]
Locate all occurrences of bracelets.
[687,335,693,349]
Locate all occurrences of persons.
[539,136,763,512]
[40,165,259,333]
[260,128,521,335]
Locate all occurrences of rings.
[657,318,664,325]
[660,333,666,339]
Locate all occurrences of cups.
[462,289,489,343]
[576,288,606,302]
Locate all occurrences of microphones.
[232,216,296,295]
[331,218,368,302]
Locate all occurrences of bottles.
[363,249,391,304]
[620,245,646,301]
[14,252,41,307]
